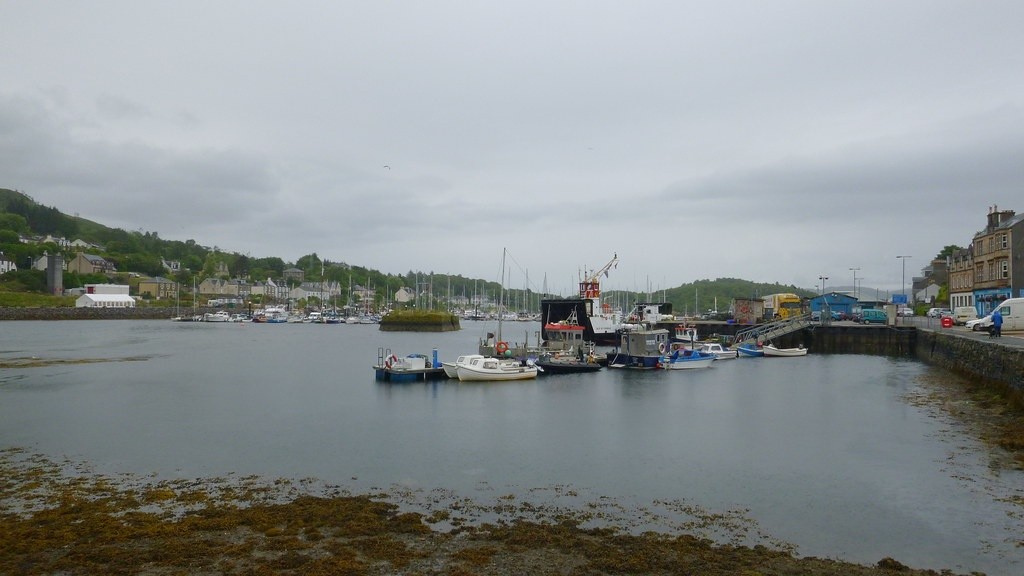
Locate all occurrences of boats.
[535,359,603,376]
[455,357,538,382]
[372,347,445,382]
[440,354,523,378]
[540,282,735,347]
[606,321,808,370]
[171,309,543,323]
[478,305,596,361]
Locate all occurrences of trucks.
[761,293,801,320]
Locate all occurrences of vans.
[952,307,978,326]
[811,309,886,325]
[979,298,1024,335]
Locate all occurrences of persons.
[991,311,1003,338]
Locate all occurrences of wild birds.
[384,166,390,171]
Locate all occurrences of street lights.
[855,278,864,300]
[819,277,829,294]
[849,268,860,301]
[896,255,912,294]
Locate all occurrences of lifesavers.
[497,342,507,353]
[659,343,666,354]
[745,305,750,311]
[555,354,559,359]
[674,344,679,350]
[741,307,747,313]
[757,340,763,346]
[595,292,599,297]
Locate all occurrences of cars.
[965,319,982,331]
[896,307,951,319]
